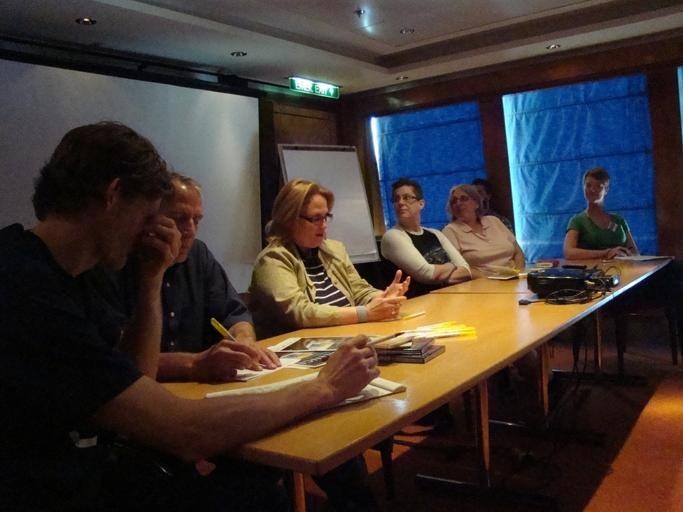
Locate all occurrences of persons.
[440,184,538,369]
[91,174,282,379]
[563,167,640,259]
[471,178,513,234]
[0,121,381,511]
[380,177,472,299]
[247,179,411,329]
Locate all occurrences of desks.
[157,253,680,511]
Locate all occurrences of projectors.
[527,265,620,299]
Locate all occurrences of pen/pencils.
[401,311,425,320]
[309,332,403,364]
[210,318,238,343]
[628,246,633,250]
[415,321,475,338]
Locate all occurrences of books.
[267,337,353,368]
[233,357,302,382]
[374,336,434,356]
[205,371,407,412]
[614,256,668,261]
[488,273,527,280]
[367,335,445,363]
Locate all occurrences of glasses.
[299,214,333,225]
[451,195,476,204]
[393,195,419,202]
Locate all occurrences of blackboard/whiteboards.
[0,58,264,296]
[276,143,381,264]
